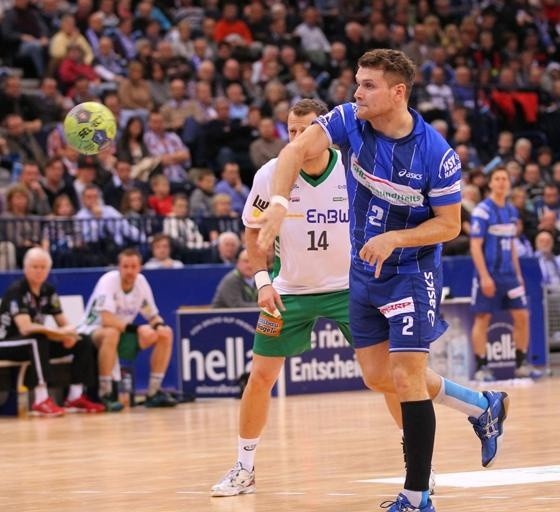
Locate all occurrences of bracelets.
[252,270,272,290]
[154,322,166,330]
[126,324,137,333]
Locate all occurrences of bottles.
[16,384,30,419]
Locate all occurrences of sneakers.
[27,389,180,418]
[467,390,510,467]
[473,369,496,380]
[511,365,543,379]
[209,461,256,497]
[385,493,436,512]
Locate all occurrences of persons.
[211,250,258,308]
[210,100,437,496]
[142,235,184,269]
[79,248,179,412]
[212,232,241,263]
[252,47,509,512]
[0,248,106,416]
[469,166,542,383]
[0,0,560,251]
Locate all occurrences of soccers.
[65,101,116,155]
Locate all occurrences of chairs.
[1,355,74,386]
